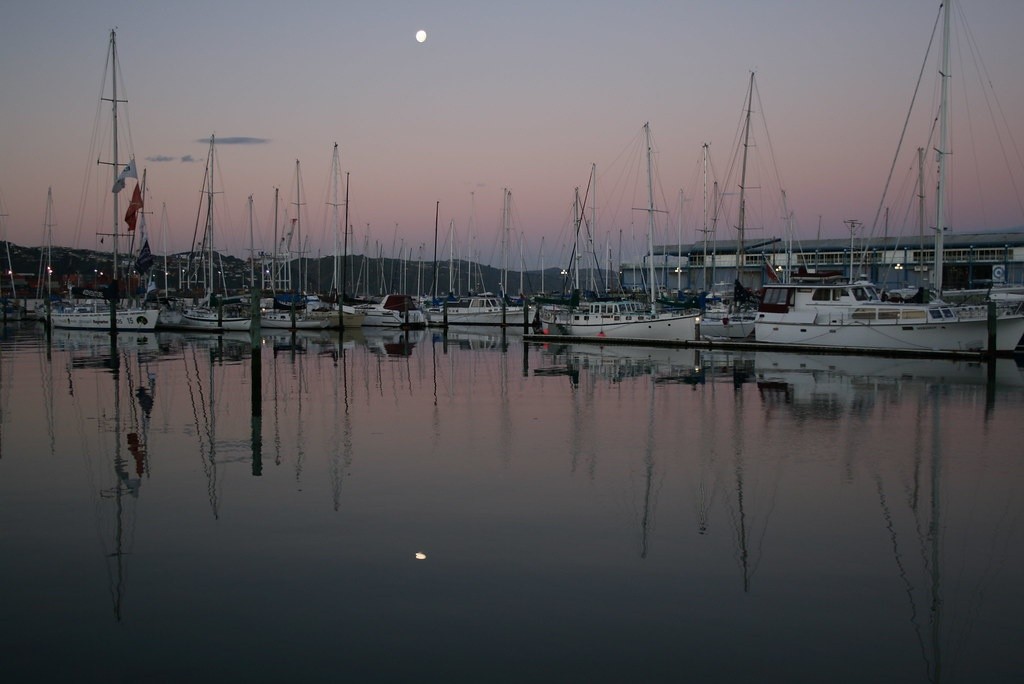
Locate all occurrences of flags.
[124,183,145,233]
[111,158,138,193]
[134,239,154,277]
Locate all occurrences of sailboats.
[755,1,1024,354]
[159,135,431,330]
[40,25,159,330]
[431,186,537,325]
[0,193,26,320]
[537,68,808,342]
